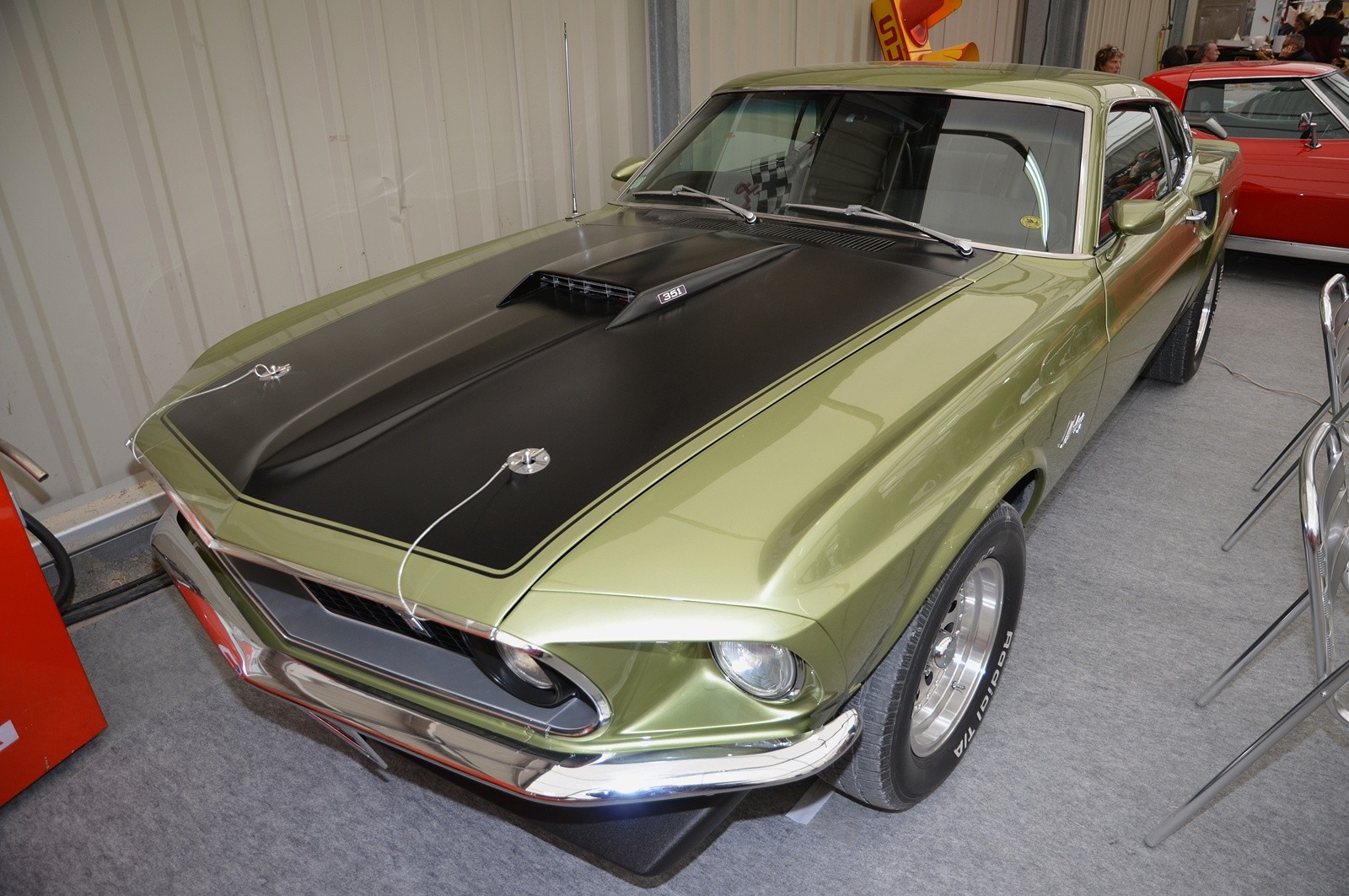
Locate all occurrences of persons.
[1094,45,1124,74]
[1294,12,1324,62]
[1162,45,1187,69]
[1193,42,1220,62]
[1257,33,1314,62]
[1317,0,1349,55]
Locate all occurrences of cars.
[1142,61,1349,265]
[123,60,1245,880]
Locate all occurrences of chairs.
[1145,274,1349,848]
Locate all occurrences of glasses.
[1103,46,1118,60]
[1282,43,1289,49]
